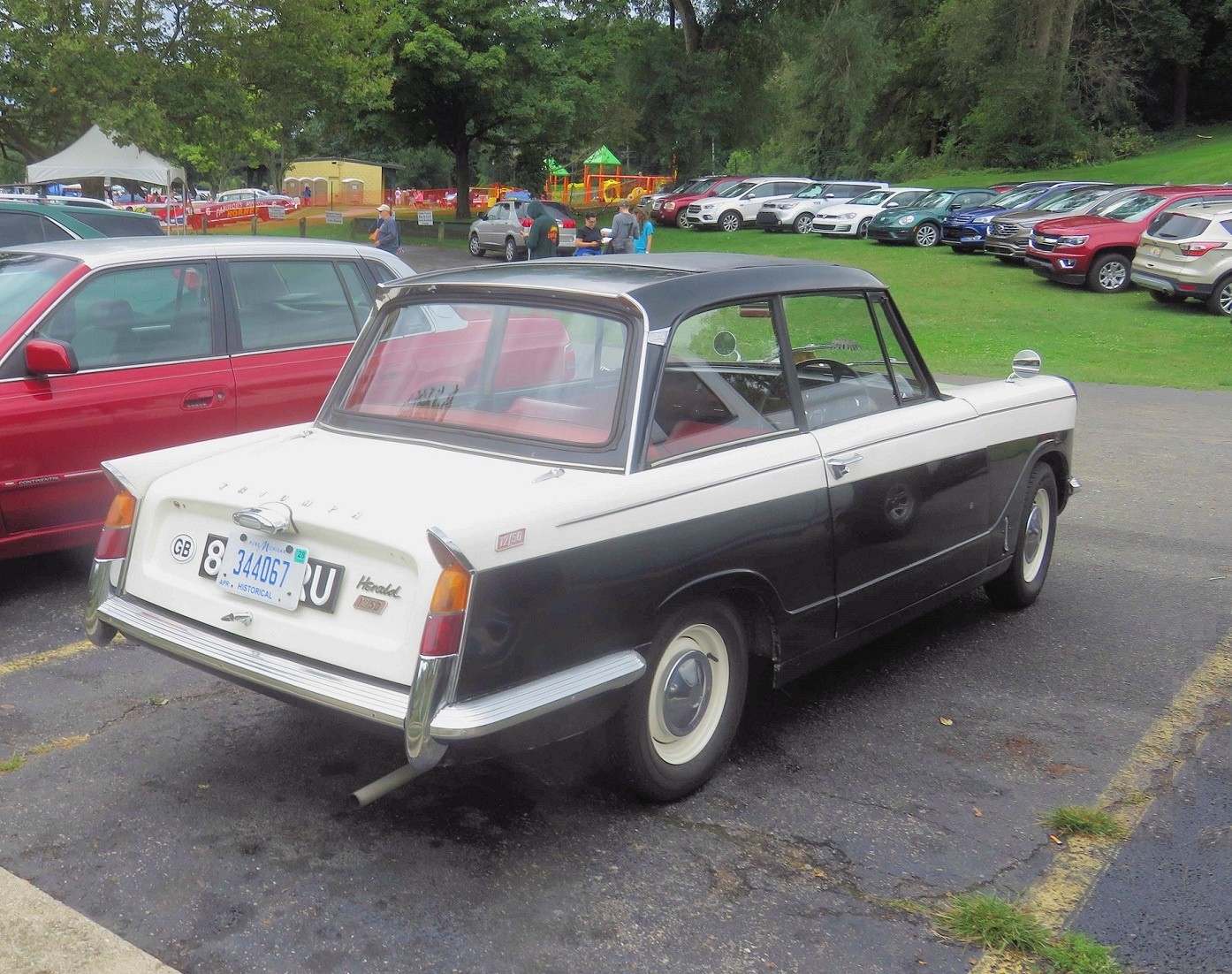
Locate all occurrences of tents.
[26,124,185,236]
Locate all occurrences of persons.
[604,200,653,254]
[520,200,560,259]
[369,204,404,254]
[575,213,604,256]
[165,192,177,203]
[241,179,290,196]
[304,186,312,206]
[14,181,22,194]
[395,187,423,207]
[150,194,158,203]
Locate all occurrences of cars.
[80,253,1079,808]
[1130,200,1232,316]
[811,188,953,240]
[0,236,576,557]
[938,181,1114,255]
[866,188,1005,247]
[985,181,1180,266]
[468,200,576,262]
[0,201,199,341]
[212,188,295,207]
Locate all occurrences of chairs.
[143,304,212,361]
[70,298,142,369]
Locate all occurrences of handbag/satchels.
[368,218,380,240]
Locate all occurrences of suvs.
[468,187,489,207]
[754,180,890,234]
[686,178,823,232]
[637,175,728,208]
[650,176,750,229]
[1025,183,1231,292]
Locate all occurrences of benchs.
[235,300,370,347]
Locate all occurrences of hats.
[396,187,400,190]
[615,200,629,212]
[376,204,390,211]
